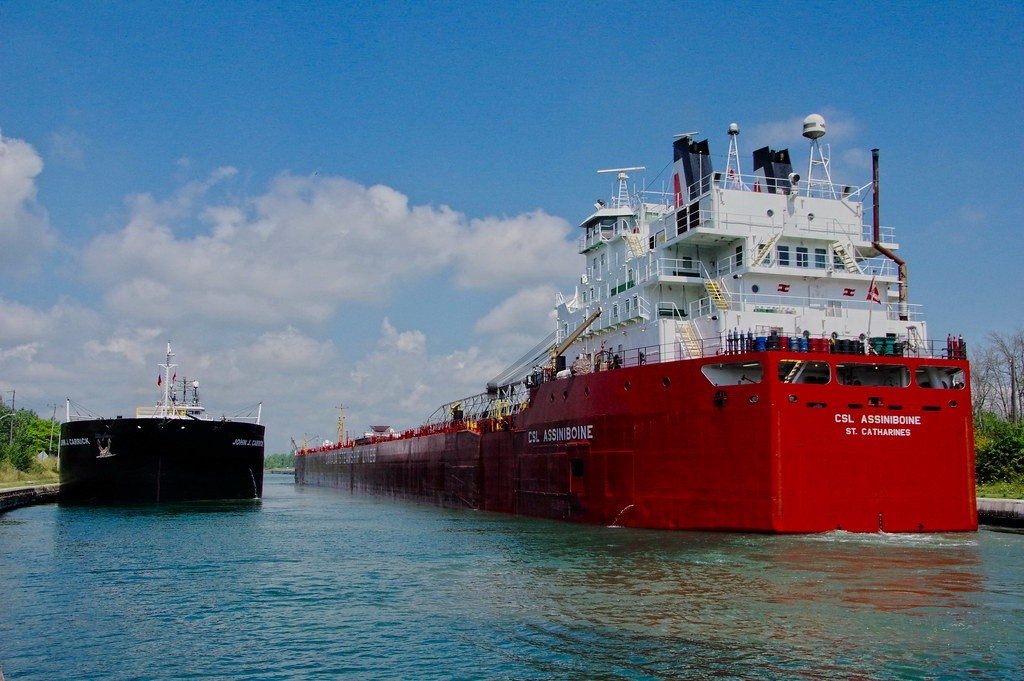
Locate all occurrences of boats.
[57,338,266,507]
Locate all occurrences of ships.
[291,111,982,540]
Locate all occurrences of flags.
[157,376,162,386]
[172,374,176,383]
[866,280,882,305]
